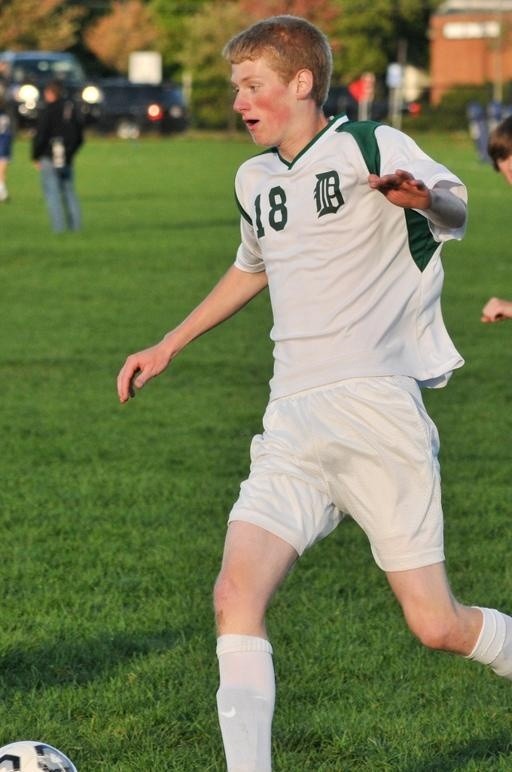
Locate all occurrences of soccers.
[0,740,77,772]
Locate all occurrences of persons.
[473,111,512,327]
[0,106,17,201]
[30,79,84,234]
[115,11,512,772]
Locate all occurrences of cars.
[324,88,422,122]
[0,52,188,136]
